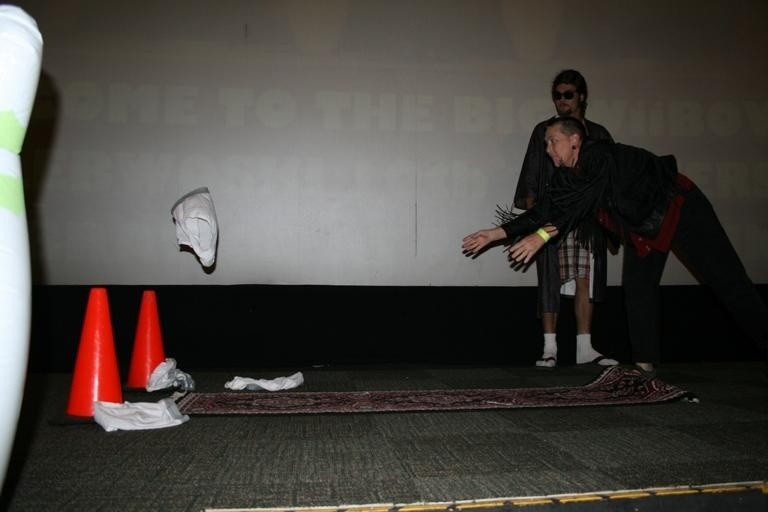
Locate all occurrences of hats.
[551,69,587,94]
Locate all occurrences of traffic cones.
[49,287,181,425]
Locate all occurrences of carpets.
[171,366,701,415]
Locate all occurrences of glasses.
[552,89,578,100]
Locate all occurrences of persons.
[510,69,619,368]
[462,118,768,376]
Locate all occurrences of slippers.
[575,355,619,368]
[536,356,558,370]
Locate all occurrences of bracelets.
[537,228,551,244]
